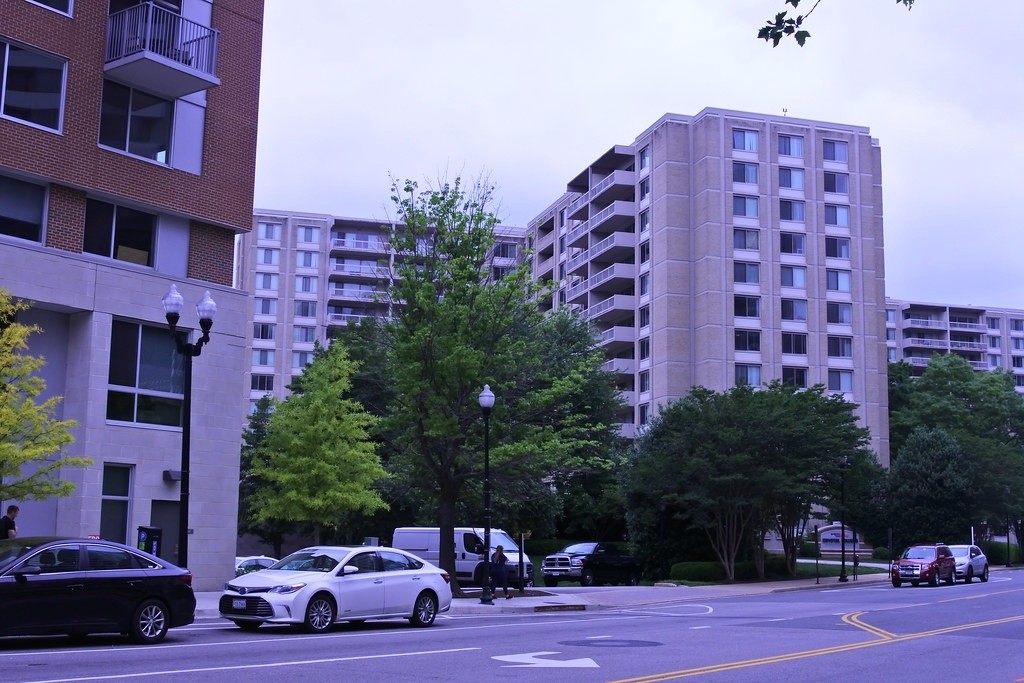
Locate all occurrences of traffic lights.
[972,527,978,545]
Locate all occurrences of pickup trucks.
[540,542,643,587]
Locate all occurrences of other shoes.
[491,594,498,599]
[506,593,514,599]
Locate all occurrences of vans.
[393,528,536,587]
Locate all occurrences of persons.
[490,545,514,599]
[0,505,19,540]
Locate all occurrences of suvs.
[892,542,958,587]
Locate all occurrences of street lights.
[837,456,849,582]
[477,383,497,606]
[158,284,219,572]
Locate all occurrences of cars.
[0,537,196,647]
[947,544,990,585]
[217,546,454,634]
